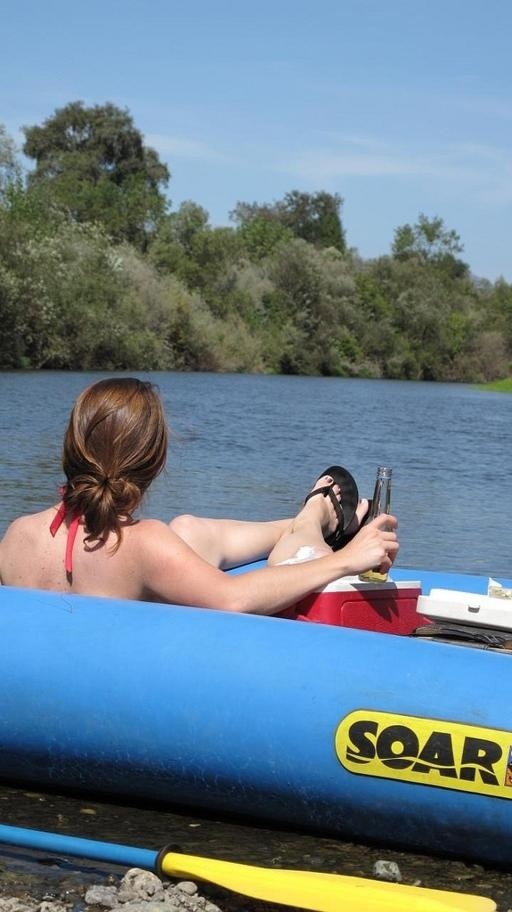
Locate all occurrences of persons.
[0,376,402,617]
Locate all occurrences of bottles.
[359,466,391,582]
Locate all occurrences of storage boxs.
[275,579,423,637]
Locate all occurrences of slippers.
[305,465,358,544]
[331,498,374,552]
